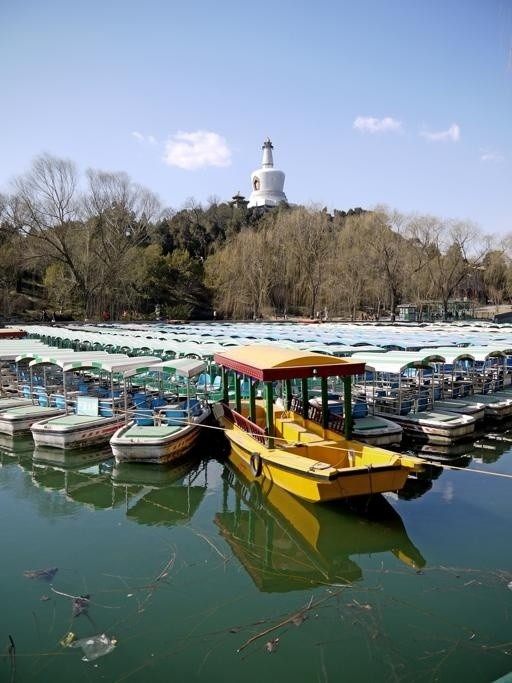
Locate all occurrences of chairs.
[324,354,511,420]
[149,369,322,401]
[9,365,202,426]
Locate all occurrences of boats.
[380,414,511,501]
[211,343,427,503]
[0,415,212,528]
[213,460,426,593]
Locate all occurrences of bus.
[397,297,475,320]
